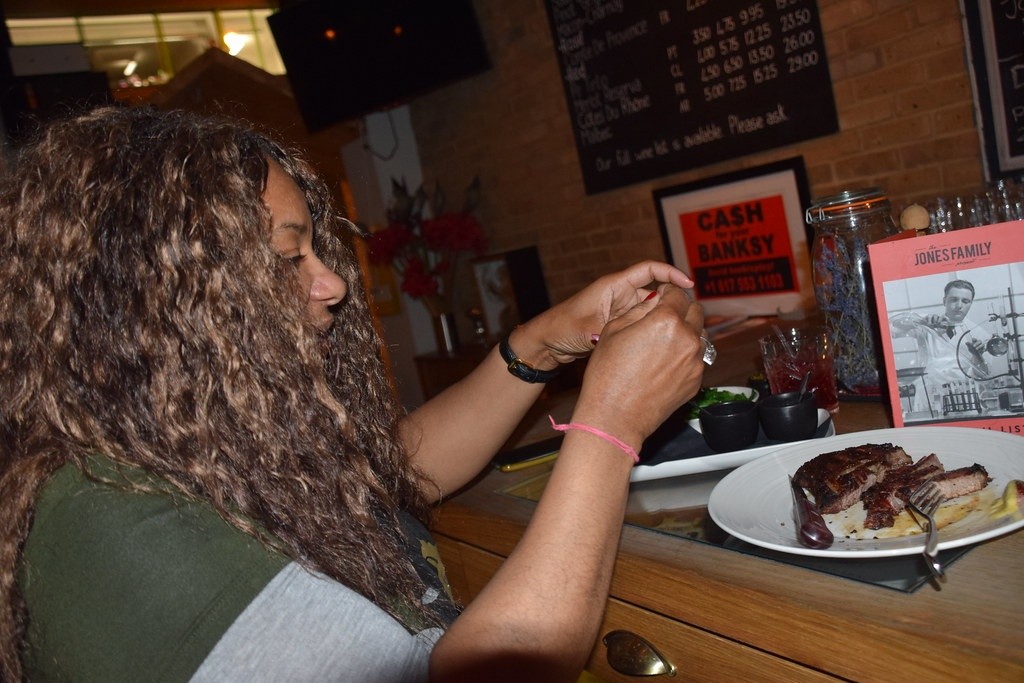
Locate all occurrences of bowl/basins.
[759,391,818,441]
[699,402,758,452]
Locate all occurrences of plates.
[629,407,836,480]
[707,428,1023,560]
[704,386,759,402]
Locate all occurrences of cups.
[924,178,1024,234]
[759,325,840,413]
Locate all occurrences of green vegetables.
[677,387,757,420]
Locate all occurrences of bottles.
[808,185,899,403]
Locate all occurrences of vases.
[400,276,454,358]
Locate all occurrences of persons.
[0,105,717,683]
[889,280,990,411]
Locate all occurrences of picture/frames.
[649,154,816,324]
[955,0,1024,184]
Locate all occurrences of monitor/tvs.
[267,0,494,136]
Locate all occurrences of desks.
[374,311,1024,683]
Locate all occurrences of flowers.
[354,177,491,303]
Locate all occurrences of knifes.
[787,474,834,549]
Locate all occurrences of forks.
[907,477,948,582]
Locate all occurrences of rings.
[697,336,716,366]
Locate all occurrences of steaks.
[792,444,990,530]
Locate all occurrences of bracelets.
[548,412,640,464]
[499,328,564,383]
